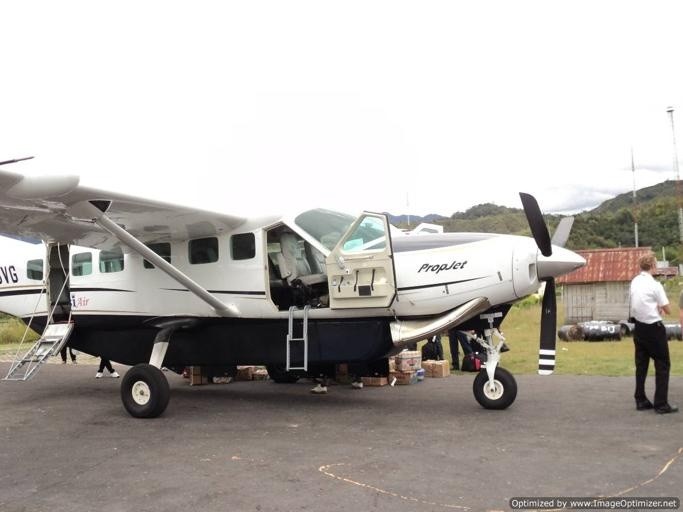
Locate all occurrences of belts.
[636,320,663,328]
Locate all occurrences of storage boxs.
[361,348,447,387]
[184,362,269,386]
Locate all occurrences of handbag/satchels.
[501,343,510,352]
[462,353,481,371]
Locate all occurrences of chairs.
[265,234,328,310]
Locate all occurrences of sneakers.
[62,361,120,378]
[351,381,363,388]
[311,384,328,393]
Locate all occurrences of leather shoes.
[636,400,654,410]
[450,366,459,371]
[654,402,678,413]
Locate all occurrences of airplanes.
[0,170,586,418]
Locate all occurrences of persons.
[628,254,678,414]
[403,318,480,370]
[59,346,79,365]
[94,356,120,378]
